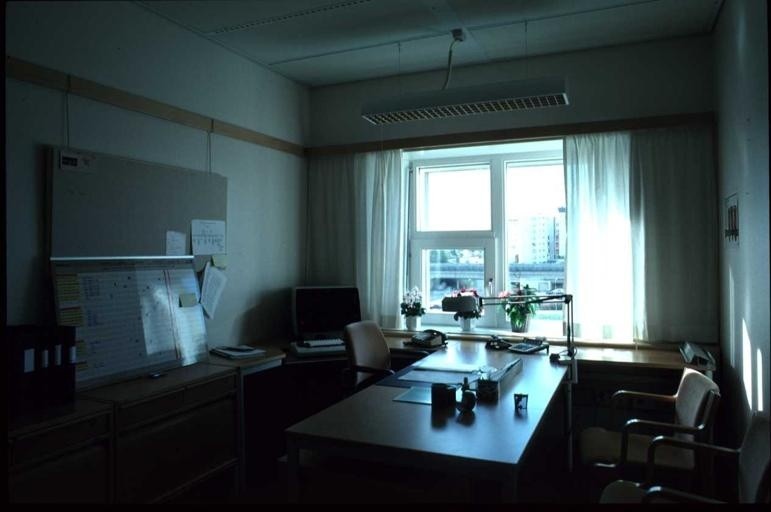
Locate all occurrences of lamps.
[360,19,570,125]
[444,289,578,385]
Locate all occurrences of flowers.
[499,283,538,316]
[451,288,483,321]
[399,285,425,315]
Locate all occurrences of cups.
[448,386,457,406]
[431,382,448,408]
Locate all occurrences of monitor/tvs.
[292,286,361,337]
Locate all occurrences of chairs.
[601,408,771,510]
[583,364,722,476]
[341,319,426,395]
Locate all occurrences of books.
[212,344,266,359]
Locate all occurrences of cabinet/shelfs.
[115,366,241,511]
[1,392,117,510]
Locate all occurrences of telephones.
[411,330,447,348]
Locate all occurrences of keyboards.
[301,339,342,346]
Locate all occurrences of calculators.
[509,337,549,353]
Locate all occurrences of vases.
[406,315,421,329]
[511,315,531,332]
[461,317,473,330]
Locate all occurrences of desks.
[281,348,569,512]
